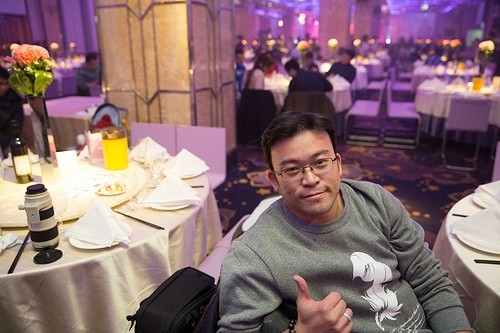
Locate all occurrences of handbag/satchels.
[132,266,216,333]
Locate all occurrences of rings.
[343,313,351,322]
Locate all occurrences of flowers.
[440,39,463,51]
[296,41,309,54]
[328,39,339,51]
[478,39,496,56]
[5,44,53,96]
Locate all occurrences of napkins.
[66,202,131,247]
[447,179,500,251]
[143,174,202,208]
[130,137,172,163]
[164,149,210,177]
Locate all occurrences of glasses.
[274,157,337,180]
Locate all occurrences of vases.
[479,53,489,76]
[27,94,60,178]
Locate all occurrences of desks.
[24,95,103,150]
[267,80,353,143]
[410,67,491,94]
[369,61,384,78]
[414,80,500,148]
[352,66,368,91]
[0,150,223,333]
[432,194,500,333]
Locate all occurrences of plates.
[130,150,170,162]
[69,222,132,250]
[457,233,500,255]
[83,152,104,164]
[3,154,40,166]
[472,192,489,209]
[161,161,207,179]
[151,203,192,209]
[95,181,128,195]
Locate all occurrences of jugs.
[472,75,482,91]
[100,126,129,172]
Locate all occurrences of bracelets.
[288,319,296,333]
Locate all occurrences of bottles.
[8,119,33,182]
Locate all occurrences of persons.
[216,109,475,333]
[284,58,333,94]
[360,35,445,71]
[76,51,102,97]
[244,54,271,90]
[324,49,356,83]
[235,34,321,72]
[0,65,24,160]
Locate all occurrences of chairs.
[129,123,228,192]
[441,95,492,170]
[238,59,422,153]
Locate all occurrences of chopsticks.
[113,209,165,231]
[7,230,30,275]
[452,213,469,217]
[148,185,205,189]
[474,259,500,264]
